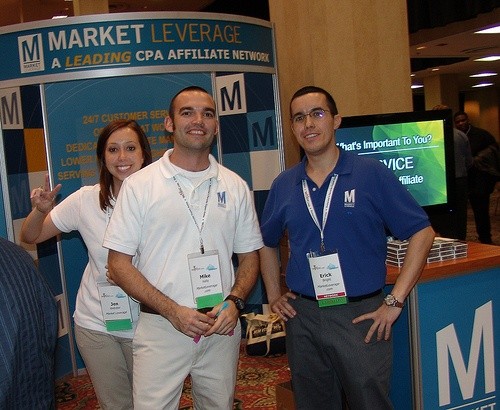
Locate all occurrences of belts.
[140,302,213,315]
[291,287,384,303]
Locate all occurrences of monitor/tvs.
[333,108,458,216]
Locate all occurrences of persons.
[101,85,264,410]
[432,105,500,245]
[0,237,59,410]
[20,118,152,410]
[258,86,435,410]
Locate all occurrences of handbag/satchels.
[239,312,286,358]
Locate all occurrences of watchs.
[224,295,245,313]
[384,294,404,308]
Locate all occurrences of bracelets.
[35,205,53,213]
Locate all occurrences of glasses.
[290,109,336,124]
